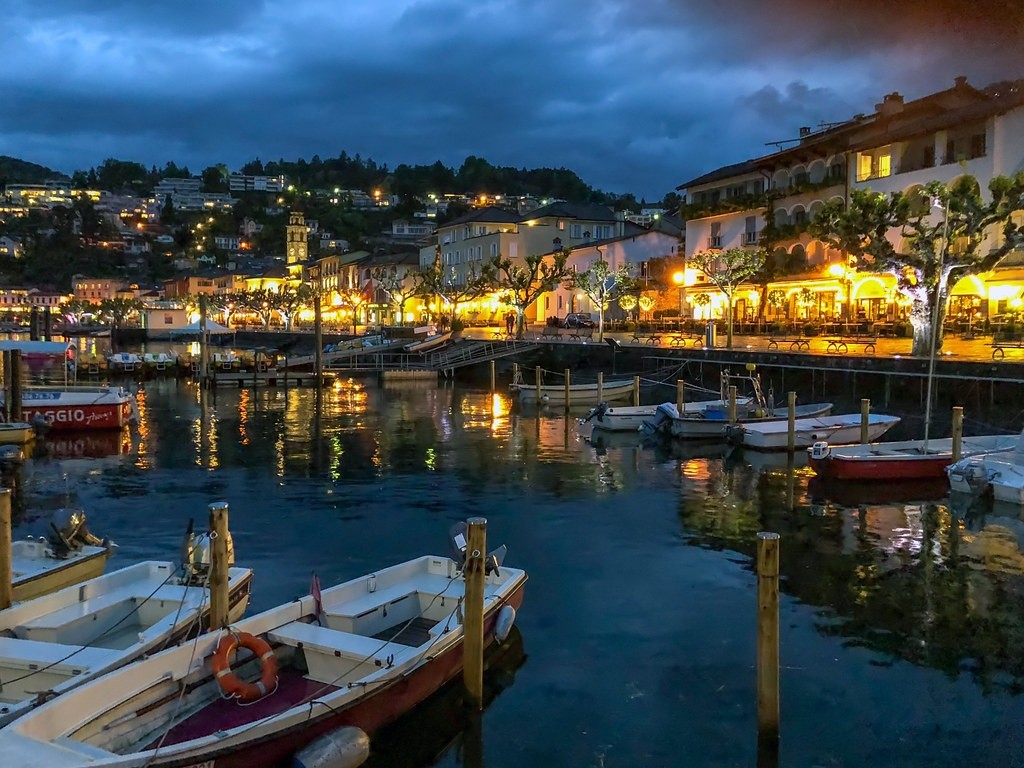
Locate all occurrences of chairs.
[115,354,125,363]
[214,353,231,370]
[158,353,173,363]
[122,352,135,371]
[144,354,157,363]
[189,356,201,371]
[130,354,142,368]
[226,353,240,363]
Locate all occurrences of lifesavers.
[213,632,277,701]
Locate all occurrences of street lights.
[831,264,854,317]
[672,272,698,316]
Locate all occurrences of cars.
[565,314,594,329]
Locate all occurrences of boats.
[584,396,756,431]
[664,402,834,436]
[805,434,1024,482]
[8,503,122,606]
[0,560,254,729]
[507,380,637,404]
[942,440,1024,504]
[0,384,140,430]
[741,412,903,446]
[74,346,275,374]
[0,554,532,768]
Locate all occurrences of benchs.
[763,337,813,350]
[984,341,1024,357]
[628,329,662,344]
[323,577,418,638]
[879,450,912,455]
[823,338,877,354]
[267,621,415,688]
[429,584,503,640]
[12,556,62,575]
[667,331,704,347]
[19,578,211,638]
[0,637,123,700]
[567,327,594,341]
[541,327,564,341]
[417,572,489,621]
